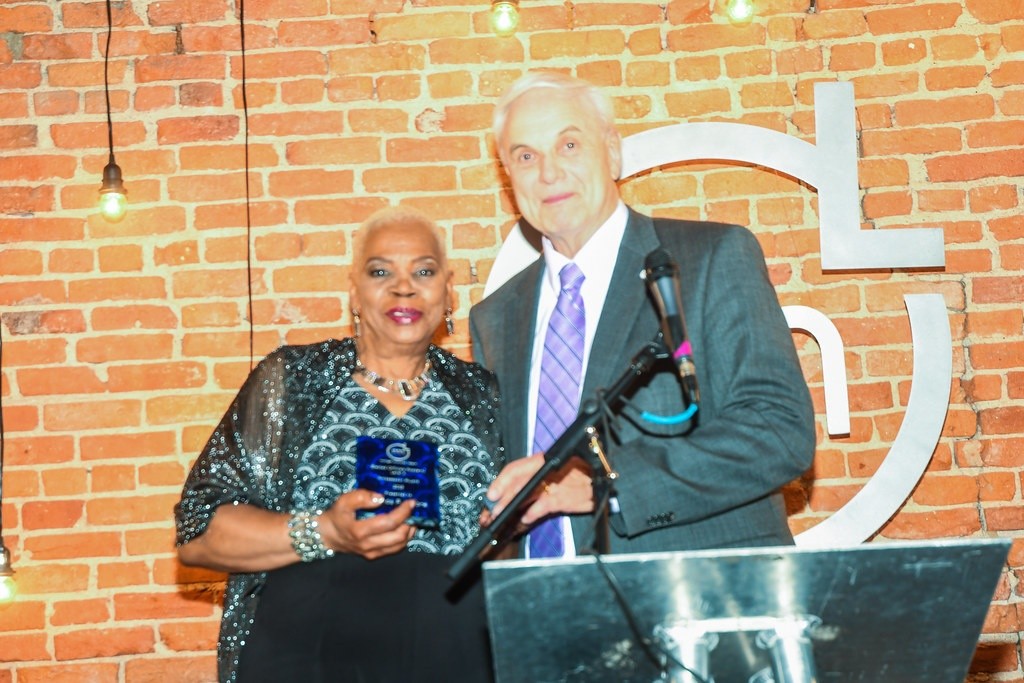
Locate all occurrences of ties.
[530,263,585,560]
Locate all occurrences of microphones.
[645,248,699,405]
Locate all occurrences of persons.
[172,207,516,683]
[468,72,816,560]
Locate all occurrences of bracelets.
[287,506,335,562]
